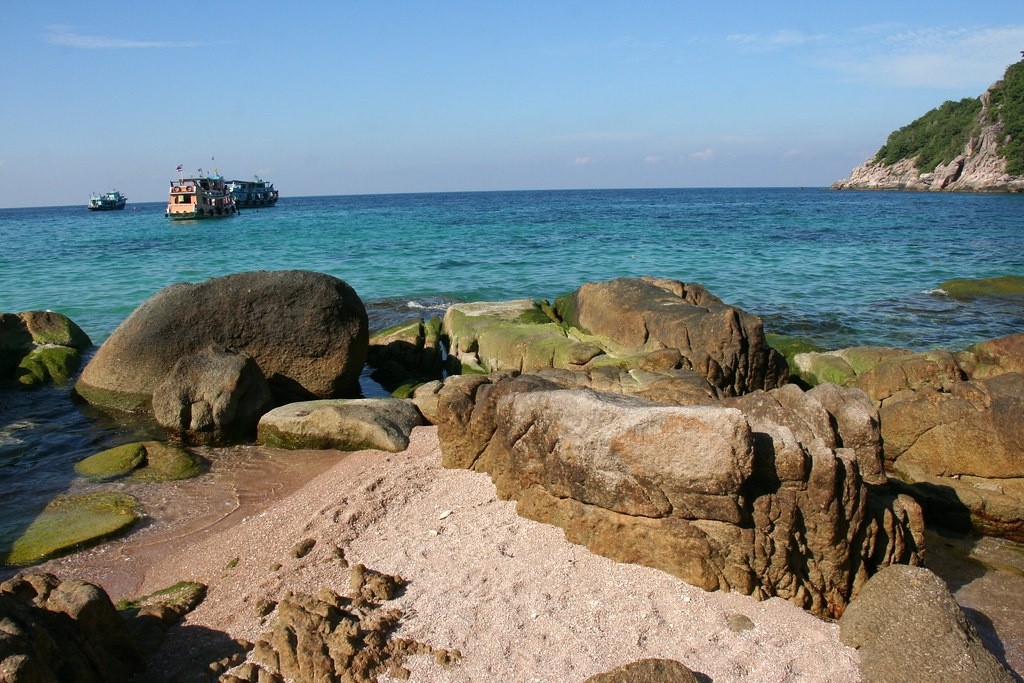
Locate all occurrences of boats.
[224,173,279,208]
[163,155,237,221]
[86,187,128,212]
